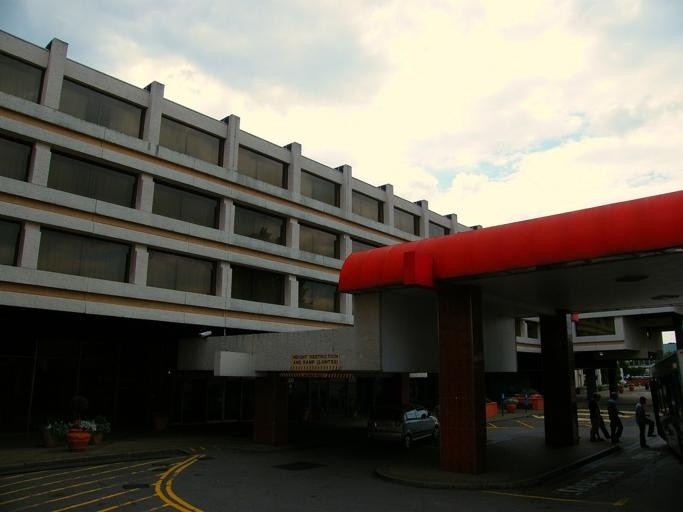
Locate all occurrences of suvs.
[366,405,440,448]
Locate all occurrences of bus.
[647,351,683,452]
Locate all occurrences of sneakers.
[598,436,619,443]
[647,434,656,437]
[641,444,649,447]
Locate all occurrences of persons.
[588,393,611,441]
[636,396,656,448]
[607,392,625,443]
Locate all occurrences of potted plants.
[63,421,93,449]
[504,398,519,413]
